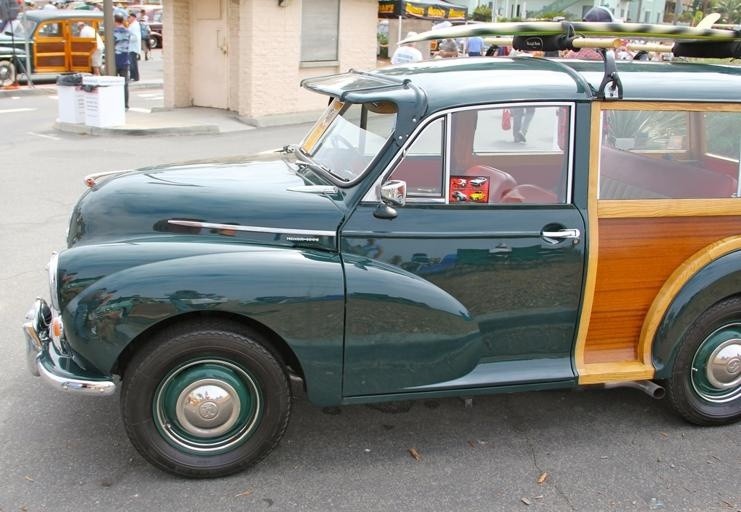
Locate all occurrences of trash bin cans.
[56,72,126,128]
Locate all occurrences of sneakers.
[515,128,527,142]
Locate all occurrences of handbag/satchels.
[502,109,512,130]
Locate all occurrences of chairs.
[463,164,557,206]
[599,143,736,199]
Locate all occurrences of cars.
[0,1,163,88]
[23,20,741,480]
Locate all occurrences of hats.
[583,8,612,22]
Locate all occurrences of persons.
[561,4,625,63]
[434,34,675,63]
[390,31,425,66]
[0,0,151,81]
[511,107,538,144]
